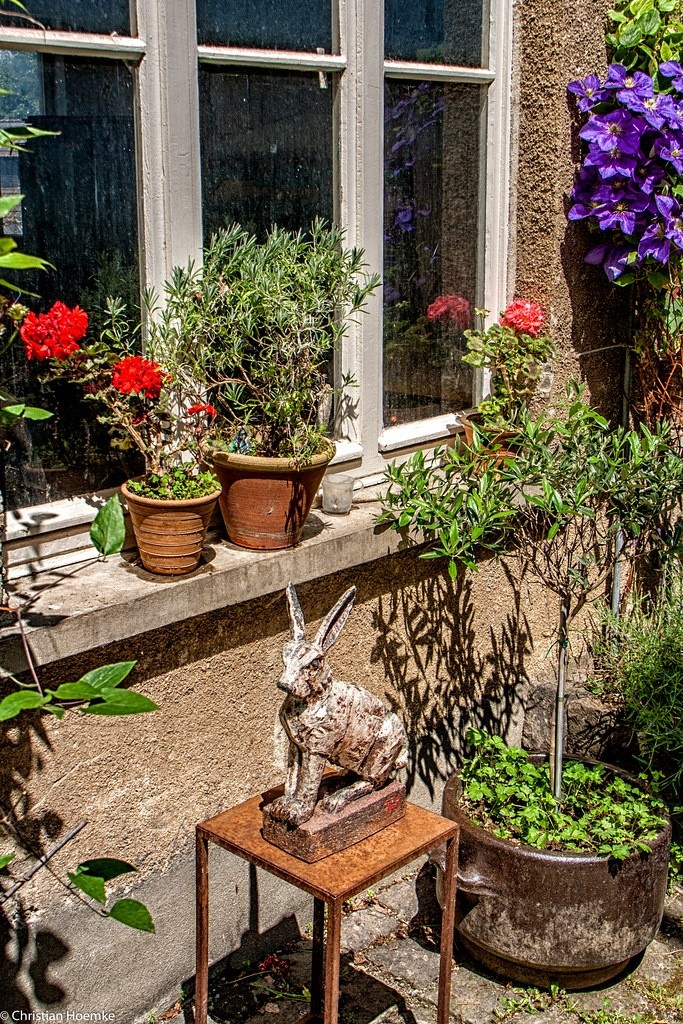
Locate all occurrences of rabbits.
[264,580,408,826]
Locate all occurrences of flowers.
[562,57,683,289]
[426,291,472,358]
[376,79,444,365]
[468,302,551,427]
[23,300,216,483]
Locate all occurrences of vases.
[120,475,217,577]
[456,410,534,478]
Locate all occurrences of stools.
[194,766,460,1022]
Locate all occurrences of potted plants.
[375,387,682,986]
[161,218,381,553]
